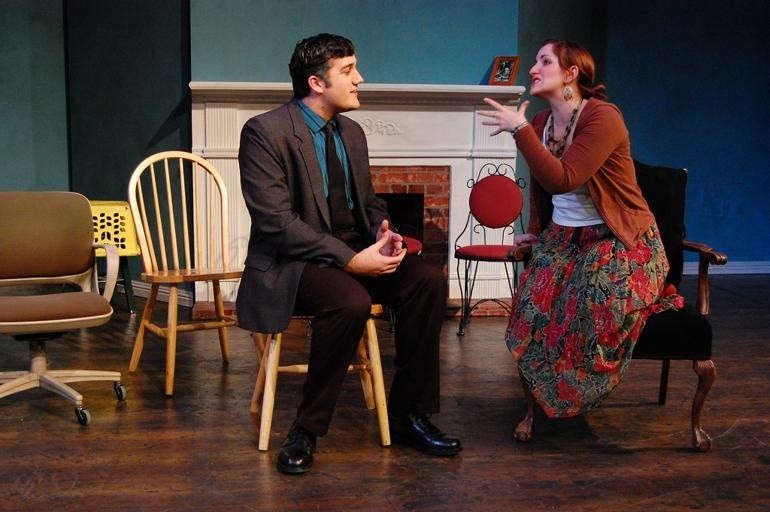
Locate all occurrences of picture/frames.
[487,56,522,85]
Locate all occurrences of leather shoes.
[390,411,462,455]
[277,420,316,474]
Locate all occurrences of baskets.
[89,200,141,257]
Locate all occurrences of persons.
[498,64,513,80]
[233,31,464,478]
[495,62,509,76]
[472,36,687,447]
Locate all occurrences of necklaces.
[546,98,585,157]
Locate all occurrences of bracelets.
[511,121,529,136]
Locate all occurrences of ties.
[323,123,355,231]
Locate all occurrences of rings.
[492,111,497,118]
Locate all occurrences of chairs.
[248,294,394,449]
[1,184,132,426]
[452,159,534,339]
[521,151,727,450]
[125,151,273,399]
